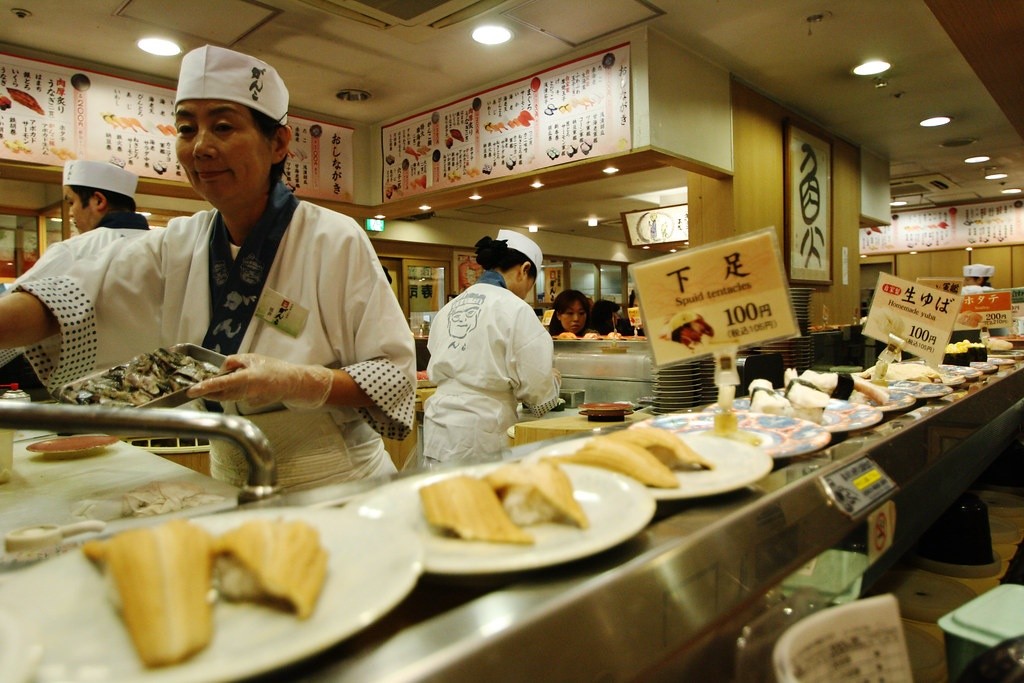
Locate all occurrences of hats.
[963,264,995,278]
[495,228,543,285]
[175,44,290,127]
[62,160,139,199]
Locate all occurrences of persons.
[13,158,151,284]
[423,229,560,471]
[548,289,590,337]
[587,299,619,334]
[0,43,415,493]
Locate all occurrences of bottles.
[851,308,860,325]
[1,383,31,403]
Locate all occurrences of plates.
[760,286,816,375]
[59,342,239,409]
[340,459,656,575]
[522,433,774,501]
[552,335,646,341]
[577,403,634,423]
[702,396,883,432]
[649,356,719,415]
[26,435,120,456]
[848,356,1016,412]
[627,411,831,457]
[0,506,425,683]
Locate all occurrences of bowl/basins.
[968,347,987,362]
[944,353,970,367]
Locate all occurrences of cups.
[0,430,15,483]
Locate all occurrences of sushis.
[942,338,987,368]
[659,312,714,349]
[957,310,981,327]
[749,362,945,424]
[417,457,587,544]
[86,512,326,667]
[559,332,623,340]
[870,308,904,336]
[570,425,714,490]
[986,339,1014,351]
